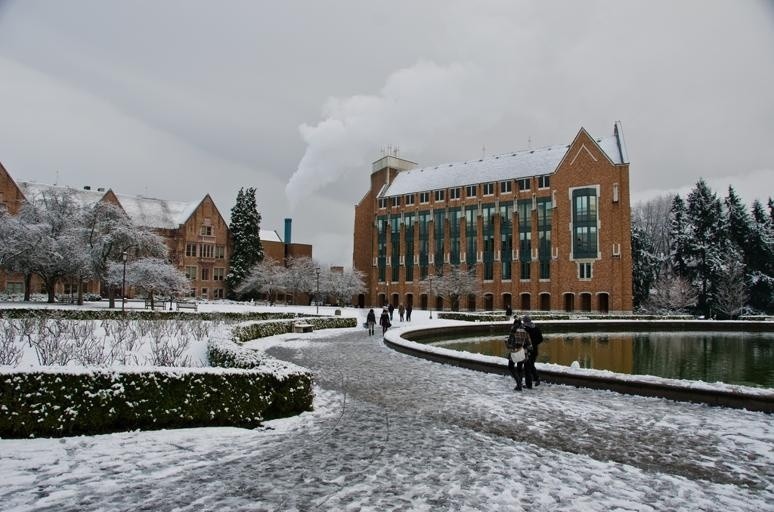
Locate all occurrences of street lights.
[315,267,320,313]
[121,251,129,311]
[428,273,434,320]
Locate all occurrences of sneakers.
[513,380,540,391]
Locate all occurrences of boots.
[368,329,374,336]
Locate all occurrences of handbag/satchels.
[509,347,526,364]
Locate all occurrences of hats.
[521,316,531,322]
[512,319,522,325]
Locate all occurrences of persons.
[380,309,390,335]
[505,315,533,392]
[521,315,544,389]
[406,304,412,321]
[388,304,394,320]
[366,309,376,335]
[506,305,512,316]
[398,303,405,322]
